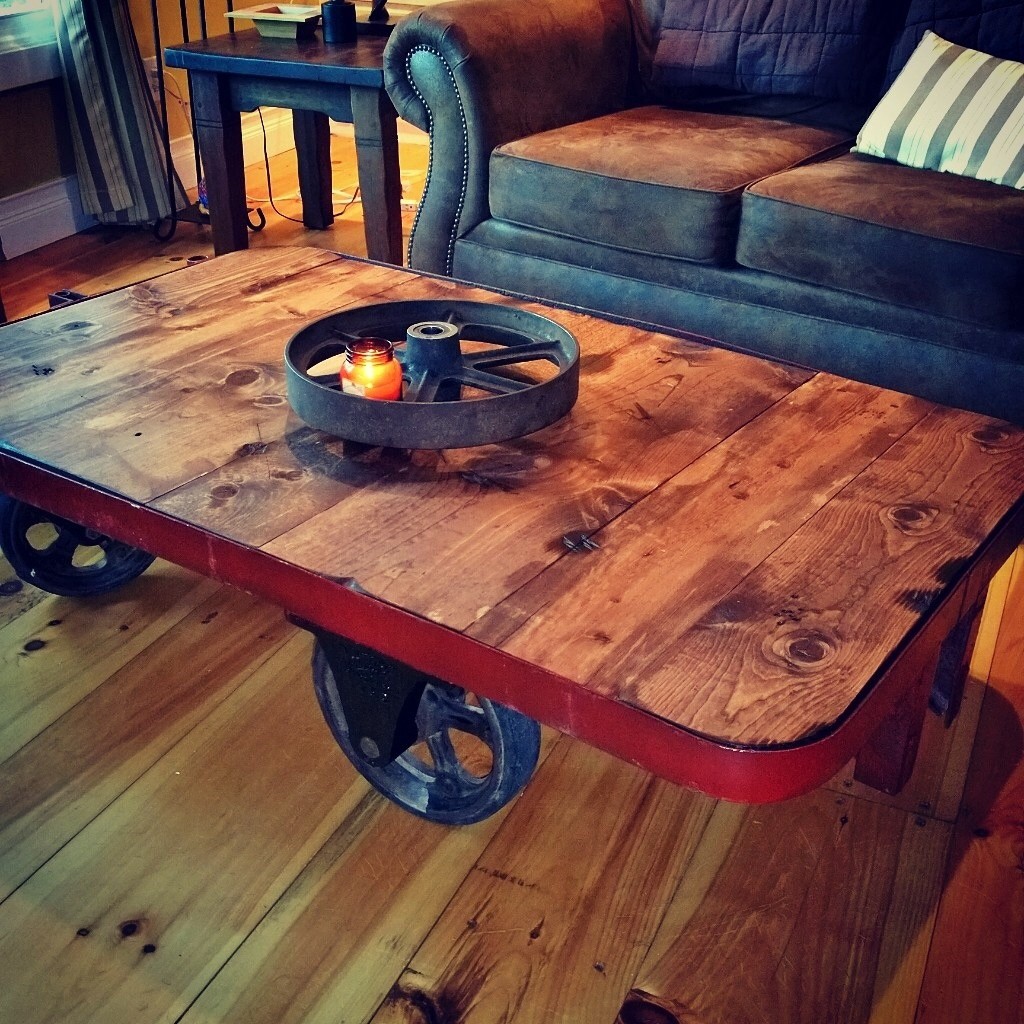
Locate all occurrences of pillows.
[848,28,1024,191]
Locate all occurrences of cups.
[321,1,357,44]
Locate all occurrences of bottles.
[339,336,403,400]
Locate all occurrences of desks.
[0,248,1024,828]
[163,9,417,267]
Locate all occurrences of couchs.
[384,1,1024,428]
